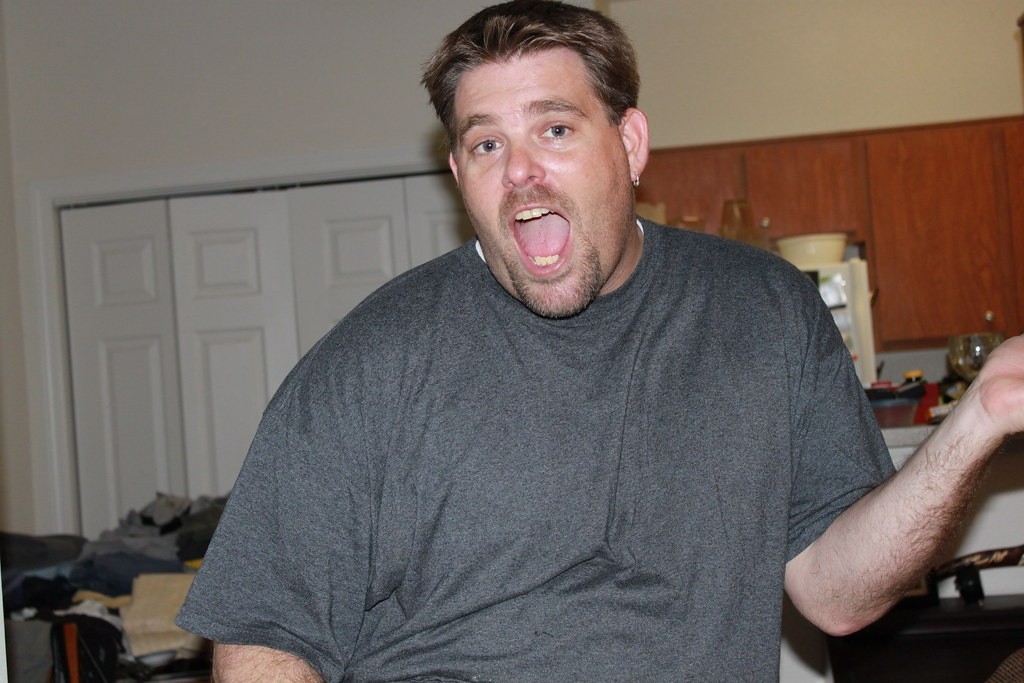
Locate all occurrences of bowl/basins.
[776,233,848,270]
[871,399,917,427]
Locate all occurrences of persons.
[176,0,1024,683]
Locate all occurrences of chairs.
[827,543,1024,683]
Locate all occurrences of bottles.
[748,217,771,252]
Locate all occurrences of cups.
[722,199,755,242]
[949,332,1004,382]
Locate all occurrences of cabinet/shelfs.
[635,230,641,231]
[48,157,474,542]
[641,114,1024,352]
